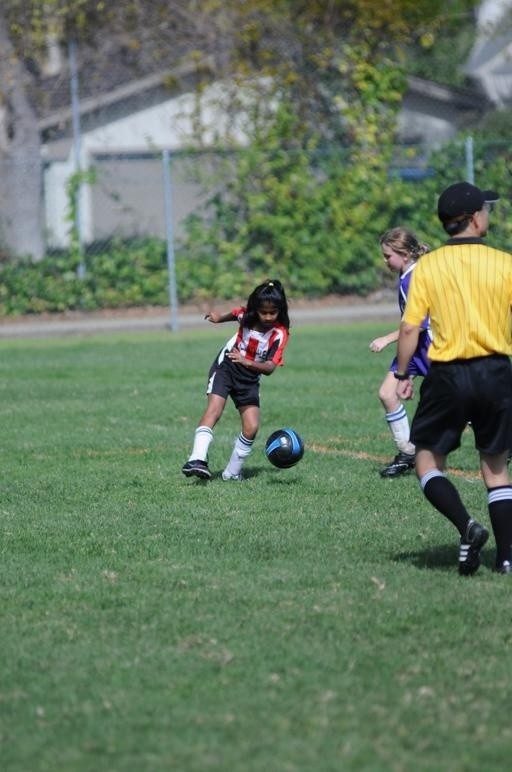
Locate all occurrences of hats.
[438,181,499,219]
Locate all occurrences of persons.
[393,182,512,576]
[181,278,289,481]
[368,228,432,479]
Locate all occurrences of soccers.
[266,428,303,468]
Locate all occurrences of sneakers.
[458,518,489,576]
[381,452,416,478]
[182,460,212,480]
[222,470,244,481]
[492,559,512,574]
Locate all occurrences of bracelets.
[393,368,410,381]
